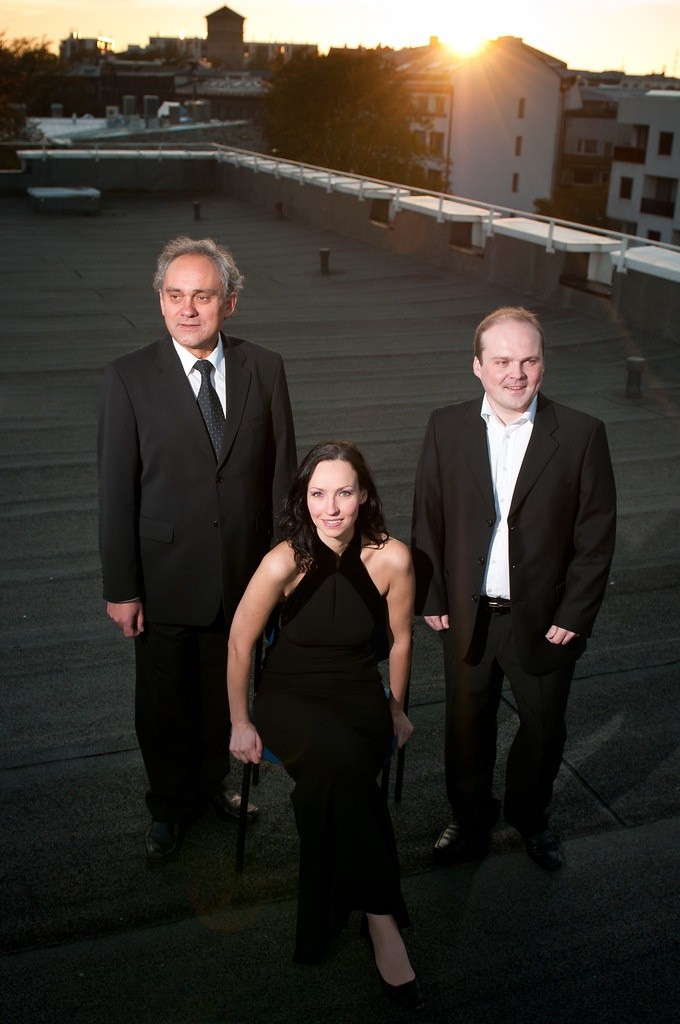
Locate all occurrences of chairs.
[236,636,415,872]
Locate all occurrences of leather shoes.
[524,830,563,871]
[143,820,178,864]
[209,789,260,823]
[358,914,425,1008]
[431,819,484,859]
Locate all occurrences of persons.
[410,304,619,874]
[98,235,303,863]
[225,438,424,1015]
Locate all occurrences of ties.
[190,361,228,463]
